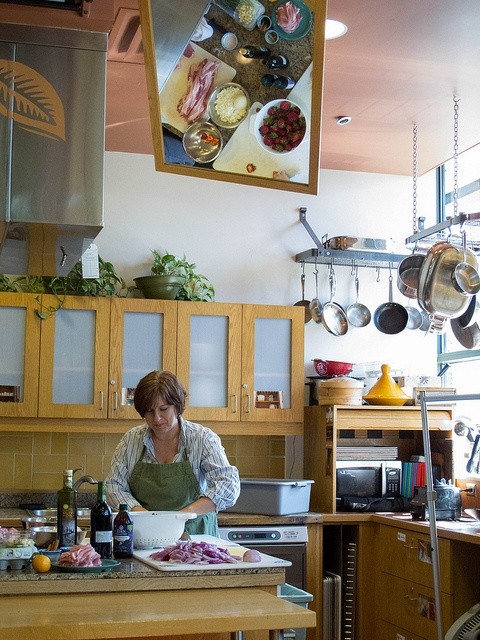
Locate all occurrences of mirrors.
[139,0,327,198]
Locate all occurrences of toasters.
[407,481,465,523]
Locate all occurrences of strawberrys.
[256,102,306,153]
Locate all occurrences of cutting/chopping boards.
[132,535,292,573]
[157,40,238,135]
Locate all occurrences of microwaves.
[333,460,403,515]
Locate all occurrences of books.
[403,462,438,499]
[338,447,398,461]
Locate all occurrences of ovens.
[217,526,308,596]
[217,524,310,633]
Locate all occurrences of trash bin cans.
[279,584,314,640]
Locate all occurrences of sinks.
[28,507,90,516]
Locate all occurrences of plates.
[221,30,238,52]
[50,556,121,573]
[269,2,312,40]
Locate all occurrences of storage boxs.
[221,478,315,516]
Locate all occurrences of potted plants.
[131,249,218,302]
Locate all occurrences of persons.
[102,370,241,538]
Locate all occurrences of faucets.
[72,468,97,494]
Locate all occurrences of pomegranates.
[31,550,51,573]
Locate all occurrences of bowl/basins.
[18,506,90,545]
[113,510,198,550]
[266,31,279,43]
[257,15,272,33]
[209,83,249,126]
[183,123,226,164]
[39,545,63,567]
[315,356,354,375]
[252,96,308,155]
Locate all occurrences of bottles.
[57,470,77,547]
[90,480,112,559]
[114,504,133,559]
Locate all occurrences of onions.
[243,550,262,562]
[150,540,241,564]
[58,544,103,567]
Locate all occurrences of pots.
[323,276,348,338]
[306,374,366,406]
[296,271,312,326]
[345,279,371,329]
[450,228,478,298]
[375,274,407,334]
[398,252,429,297]
[402,304,421,330]
[310,274,325,326]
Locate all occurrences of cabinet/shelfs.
[305,403,454,514]
[176,301,305,424]
[0,291,43,419]
[371,515,480,640]
[0,20,108,238]
[38,294,179,420]
